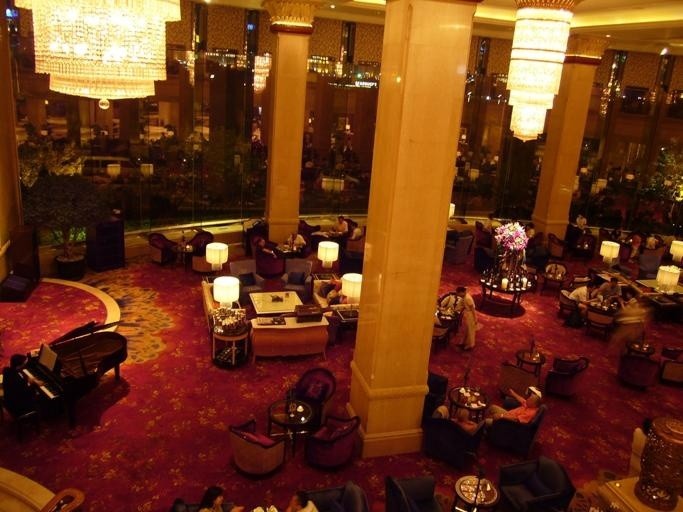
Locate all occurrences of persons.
[332,216,349,235]
[628,417,651,478]
[331,274,347,304]
[197,486,244,512]
[431,385,543,434]
[350,223,362,241]
[441,287,468,314]
[3,354,45,418]
[510,214,659,323]
[287,232,306,248]
[456,287,477,351]
[286,490,319,512]
[257,238,277,259]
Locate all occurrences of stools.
[4,400,40,441]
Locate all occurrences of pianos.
[16,319,127,428]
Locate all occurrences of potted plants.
[21,172,102,281]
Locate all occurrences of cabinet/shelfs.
[248,316,330,364]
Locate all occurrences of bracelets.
[272,251,274,254]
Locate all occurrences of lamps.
[341,272,363,313]
[17,118,501,198]
[175,40,195,89]
[503,1,578,146]
[213,275,240,310]
[246,52,273,96]
[12,1,182,110]
[204,242,228,277]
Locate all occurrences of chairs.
[422,373,448,425]
[445,202,541,273]
[286,367,337,430]
[228,418,287,477]
[426,412,486,470]
[228,252,314,300]
[486,394,546,454]
[170,500,236,511]
[495,358,539,403]
[302,414,361,470]
[384,475,443,510]
[498,456,576,510]
[296,485,369,511]
[541,219,682,338]
[146,224,213,274]
[241,215,357,275]
[545,355,589,398]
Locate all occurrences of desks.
[210,332,247,365]
[514,347,545,378]
[265,398,314,457]
[477,275,530,316]
[448,384,489,423]
[452,474,498,511]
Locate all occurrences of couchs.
[200,280,241,330]
[311,279,351,310]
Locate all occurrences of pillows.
[317,280,346,305]
